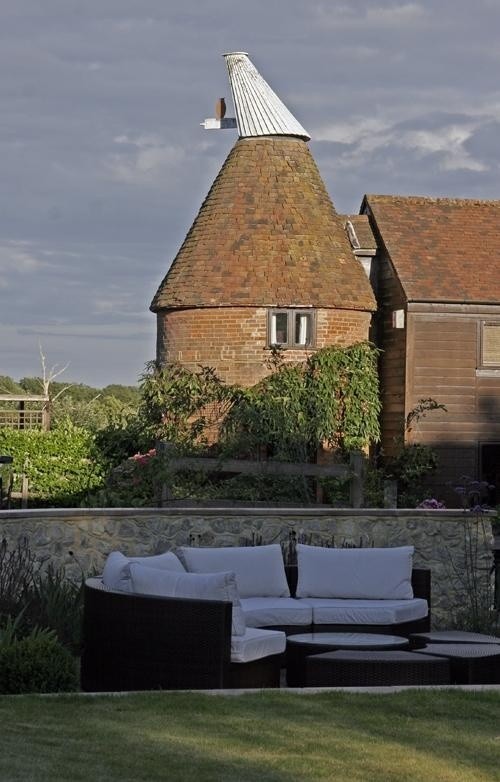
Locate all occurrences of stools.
[411,629,500,644]
[307,647,450,685]
[413,641,500,685]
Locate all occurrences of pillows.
[129,551,188,574]
[180,543,291,597]
[102,551,135,592]
[129,564,245,636]
[295,543,415,600]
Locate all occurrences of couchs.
[83,542,431,690]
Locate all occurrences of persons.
[415,489,439,510]
